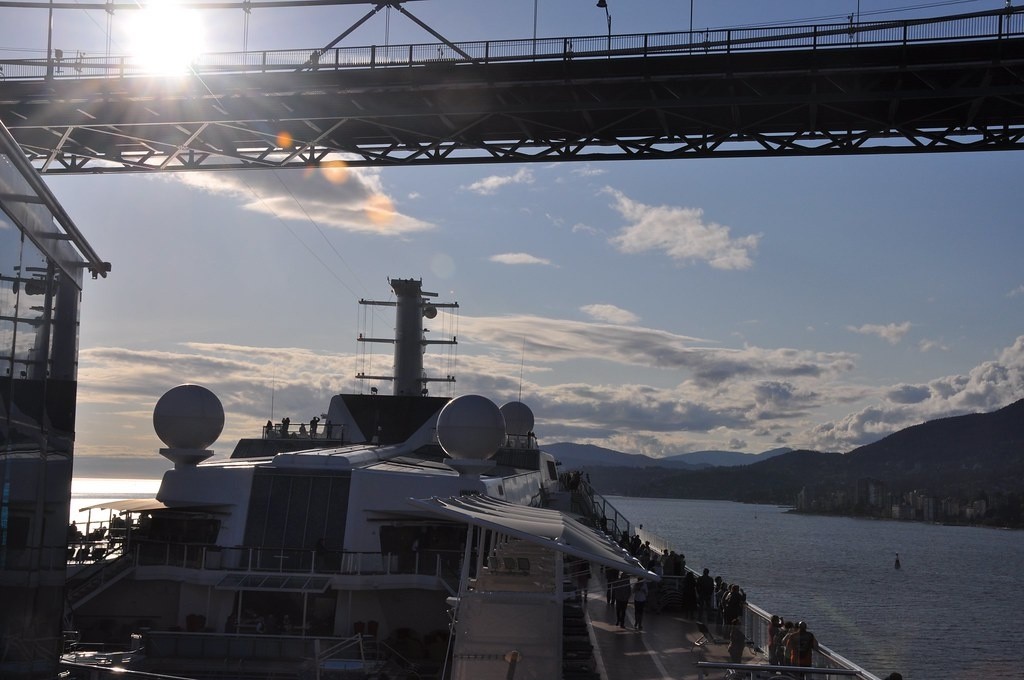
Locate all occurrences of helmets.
[798,621,806,628]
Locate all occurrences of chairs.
[691,622,756,662]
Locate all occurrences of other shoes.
[634,623,643,629]
[607,603,614,606]
[616,621,625,628]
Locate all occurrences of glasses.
[736,622,741,625]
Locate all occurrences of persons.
[559,470,592,491]
[265,416,333,441]
[768,615,818,680]
[599,515,747,663]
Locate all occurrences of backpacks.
[773,631,788,645]
